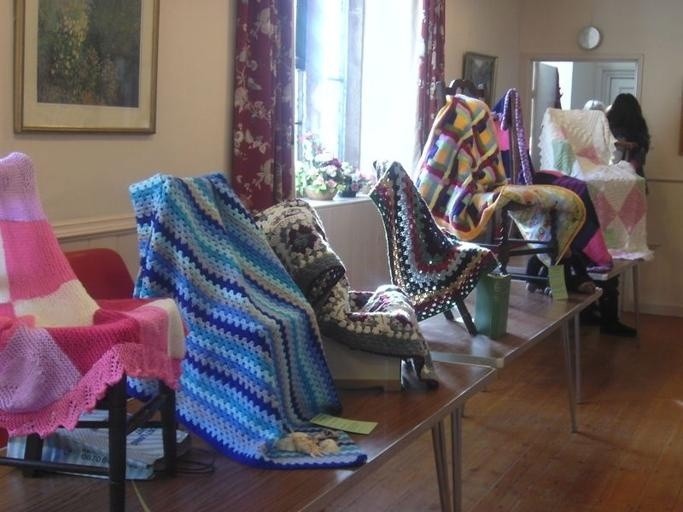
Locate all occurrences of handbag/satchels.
[6,408,216,481]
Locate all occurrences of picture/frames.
[463,52,498,110]
[11,2,158,135]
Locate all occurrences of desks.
[499,244,661,401]
[0,267,602,511]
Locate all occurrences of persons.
[579,93,650,338]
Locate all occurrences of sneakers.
[600,321,637,337]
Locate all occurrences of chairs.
[491,88,613,275]
[135,172,342,436]
[260,200,402,394]
[62,250,134,300]
[0,152,179,512]
[435,79,558,276]
[373,161,497,337]
[543,107,647,255]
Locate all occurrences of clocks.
[576,25,600,50]
